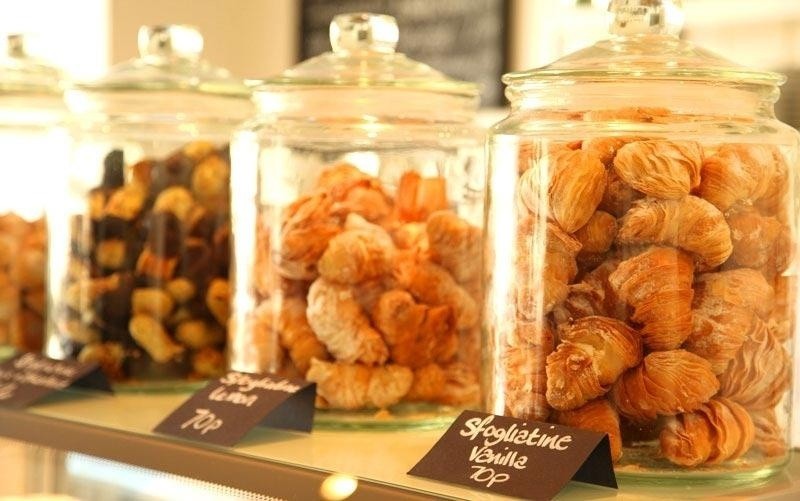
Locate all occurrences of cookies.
[51,140,237,382]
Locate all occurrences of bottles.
[230,12,499,428]
[502,0,799,491]
[0,26,266,383]
